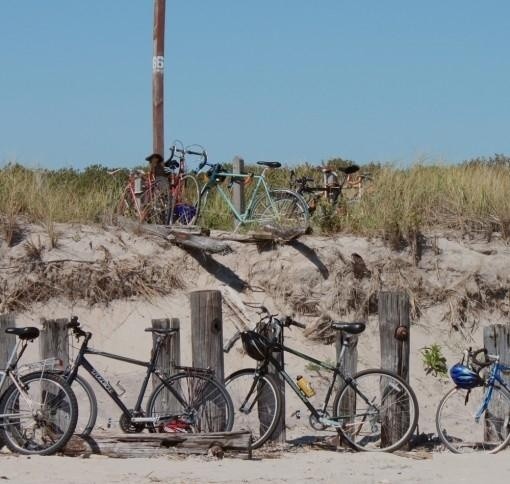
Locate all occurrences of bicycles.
[5,315,234,447]
[119,139,372,242]
[0,327,76,455]
[436,344,509,454]
[223,305,419,452]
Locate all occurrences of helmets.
[450,362,479,388]
[240,330,270,360]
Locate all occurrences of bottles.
[297,375,316,398]
[108,378,125,396]
